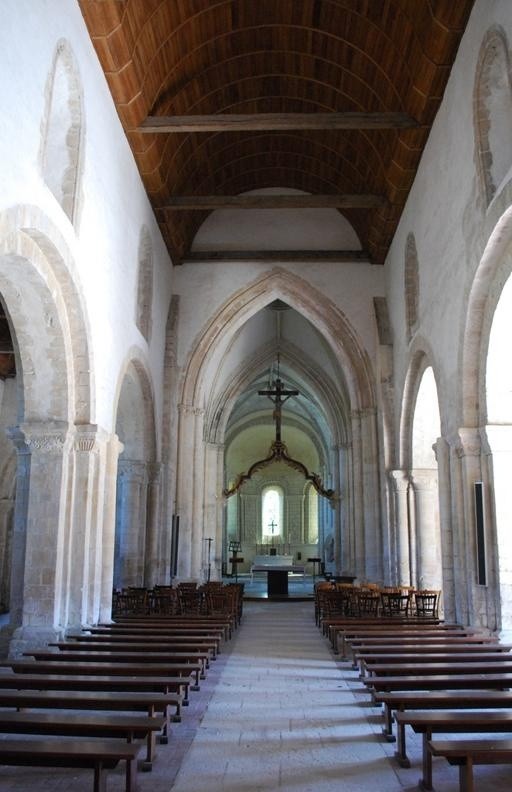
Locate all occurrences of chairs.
[114,582,245,629]
[314,583,442,627]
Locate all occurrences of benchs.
[0,622,225,792]
[330,624,512,792]
